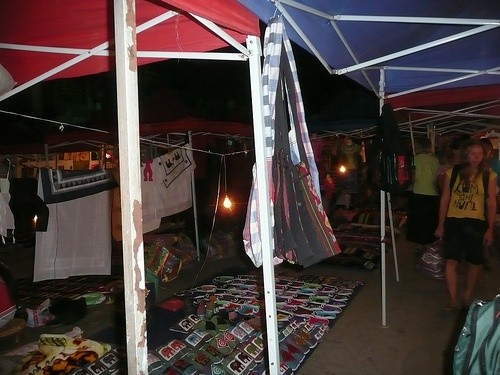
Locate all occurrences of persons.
[435,140,497,315]
[406,137,500,272]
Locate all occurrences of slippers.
[70,303,330,375]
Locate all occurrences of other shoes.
[440,305,456,312]
[414,247,425,256]
[179,270,364,320]
